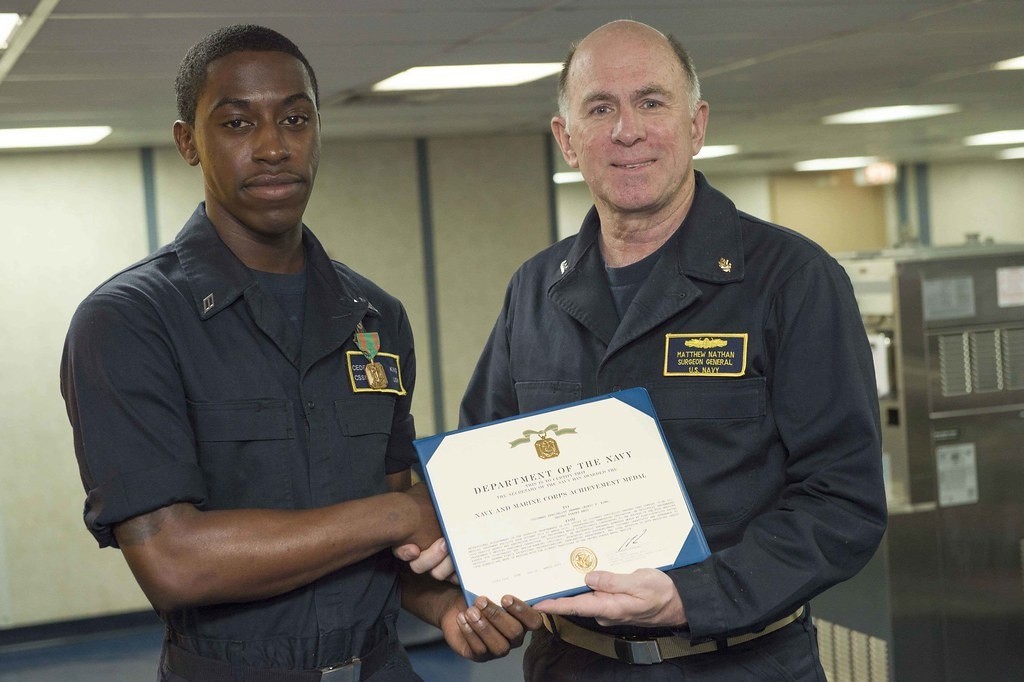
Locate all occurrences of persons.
[59,23,545,682]
[388,18,887,682]
